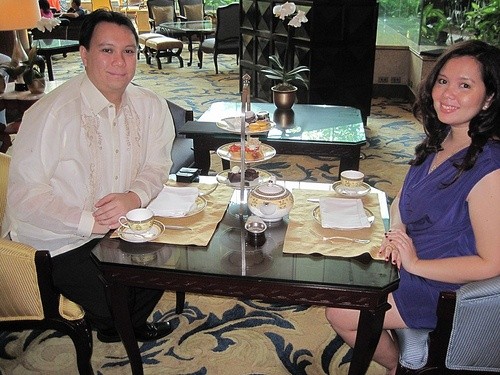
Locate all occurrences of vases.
[27,78,45,94]
[271,86,298,111]
[273,109,295,129]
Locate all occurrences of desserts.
[227,166,260,183]
[229,138,264,159]
[245,110,271,130]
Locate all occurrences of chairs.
[391,276,500,375]
[132,83,210,174]
[147,0,240,74]
[0,150,96,375]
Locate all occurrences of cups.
[341,170,365,194]
[118,208,154,234]
[244,216,266,248]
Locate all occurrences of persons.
[0,0,180,342]
[324,38,500,375]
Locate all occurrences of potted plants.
[204,0,237,24]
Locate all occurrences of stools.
[138,32,164,64]
[146,37,184,70]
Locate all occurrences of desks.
[0,80,69,124]
[158,20,216,67]
[89,100,401,375]
[31,39,80,81]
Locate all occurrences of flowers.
[4,16,61,86]
[260,1,311,91]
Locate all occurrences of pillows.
[151,6,174,26]
[183,3,203,21]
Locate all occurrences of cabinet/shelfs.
[239,0,380,117]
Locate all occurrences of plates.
[148,194,207,218]
[332,182,371,198]
[312,206,375,225]
[118,219,166,243]
[216,115,276,188]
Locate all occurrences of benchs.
[59,14,90,53]
[30,18,71,60]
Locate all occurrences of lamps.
[0,0,41,92]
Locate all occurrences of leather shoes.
[97,320,174,343]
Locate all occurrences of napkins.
[147,185,199,217]
[318,196,371,229]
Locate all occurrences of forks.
[310,231,370,244]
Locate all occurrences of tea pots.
[247,179,294,222]
[262,217,287,254]
